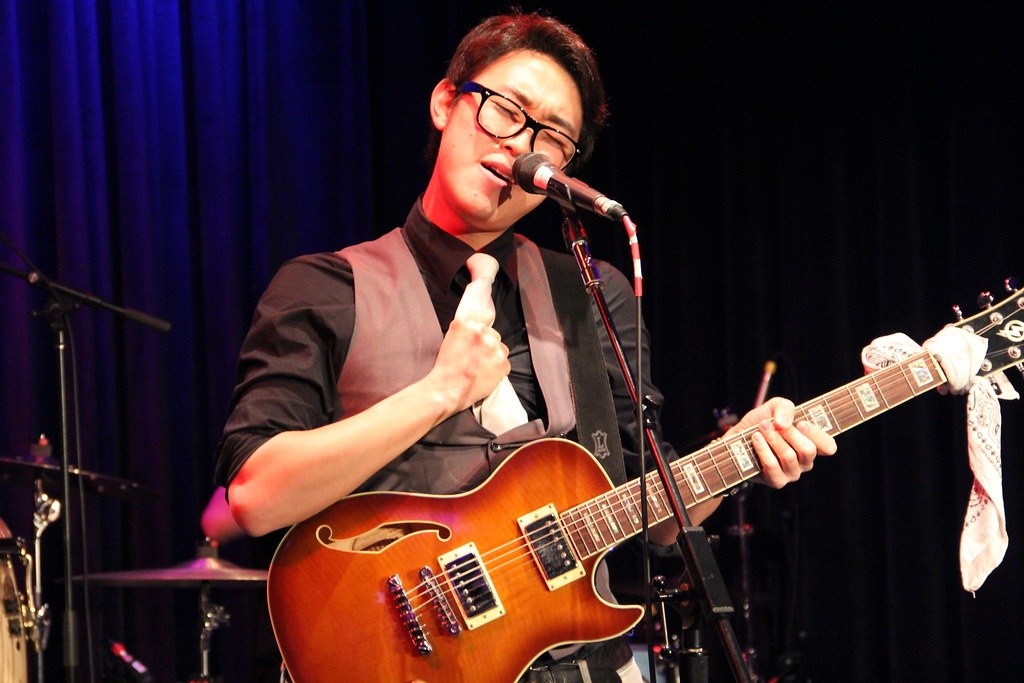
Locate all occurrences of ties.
[453,253,529,438]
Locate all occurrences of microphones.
[512,152,629,224]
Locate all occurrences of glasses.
[459,78,582,173]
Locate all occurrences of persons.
[211,11,840,683]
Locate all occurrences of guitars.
[267,276,1024,683]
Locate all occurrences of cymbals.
[0,434,140,494]
[53,538,269,589]
